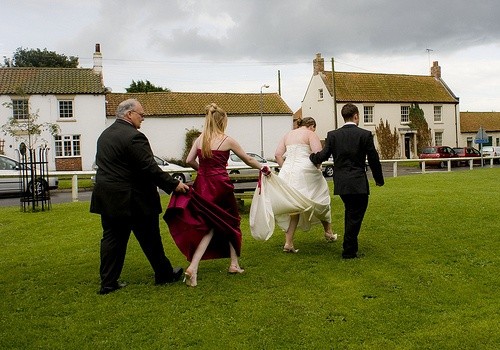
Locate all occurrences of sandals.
[283,244,299,254]
[324,232,338,243]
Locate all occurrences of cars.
[0,154,59,198]
[451,146,486,167]
[225,151,280,183]
[320,154,334,179]
[90,153,193,190]
[418,145,460,169]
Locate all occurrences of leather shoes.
[99,283,129,295]
[155,267,184,285]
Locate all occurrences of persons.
[163,103,272,287]
[309,103,384,259]
[249,117,337,252]
[90,99,189,295]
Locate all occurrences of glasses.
[131,110,144,119]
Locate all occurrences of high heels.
[228,264,245,277]
[182,266,197,288]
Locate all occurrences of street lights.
[260,84,271,163]
